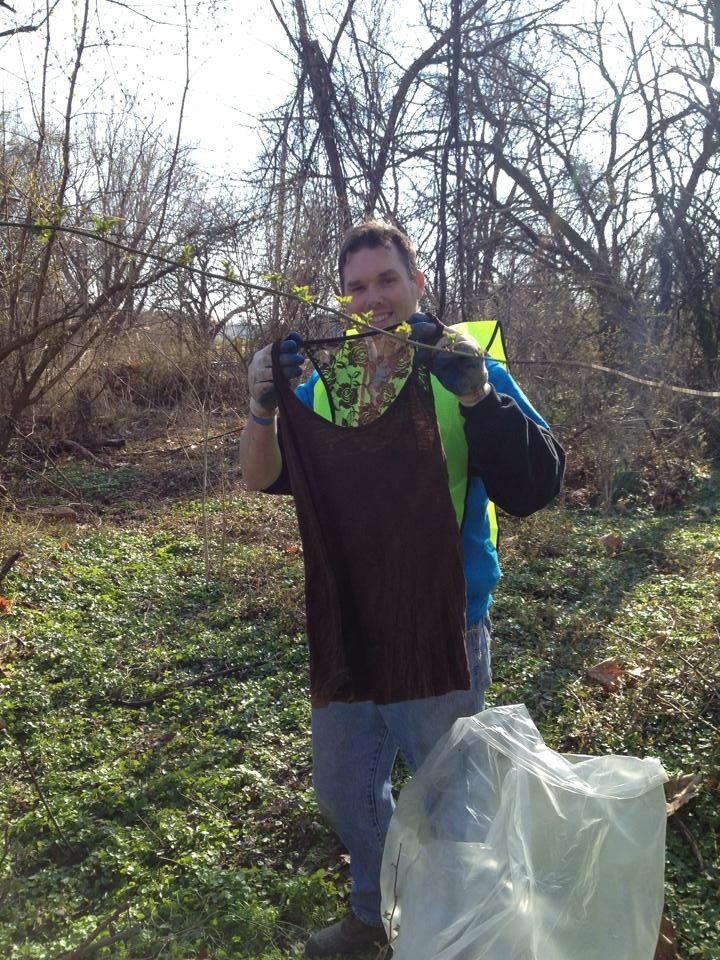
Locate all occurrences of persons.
[241,222,567,960]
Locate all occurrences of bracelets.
[250,412,273,425]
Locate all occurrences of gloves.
[411,312,489,397]
[246,332,305,425]
